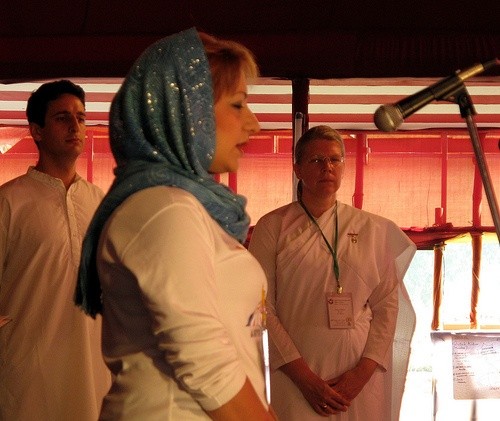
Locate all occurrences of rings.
[322,404,328,410]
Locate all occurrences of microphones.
[373,58,498,135]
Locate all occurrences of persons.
[1,80,113,421]
[247,123,404,420]
[88,28,279,421]
